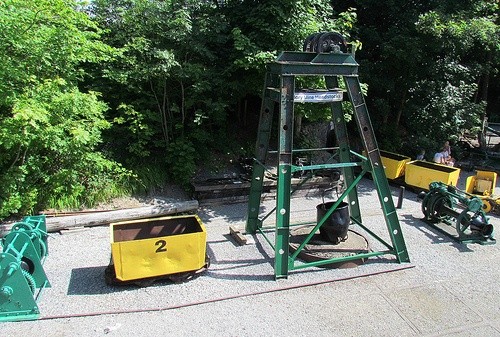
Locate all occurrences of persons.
[434,139,456,168]
